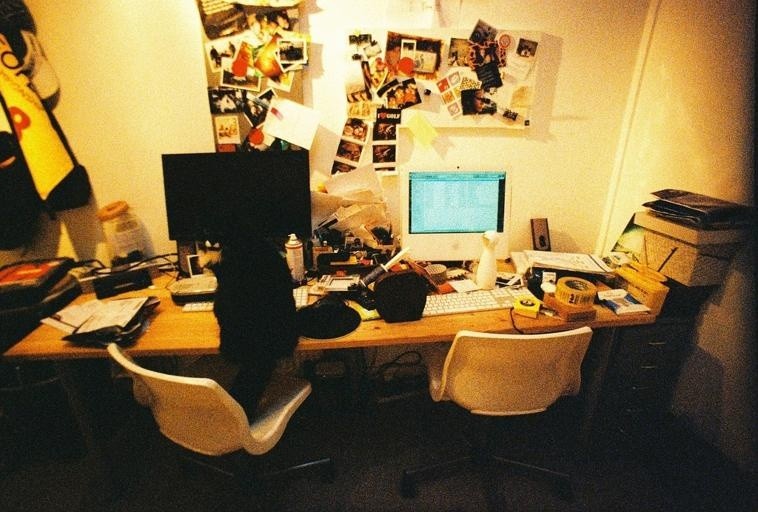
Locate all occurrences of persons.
[209,45,220,68]
[228,42,236,57]
[360,23,531,116]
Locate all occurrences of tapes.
[426,265,447,284]
[601,274,615,284]
[556,277,597,307]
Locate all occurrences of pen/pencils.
[643,234,678,272]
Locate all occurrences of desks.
[4,258,657,511]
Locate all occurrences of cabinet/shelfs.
[574,311,694,465]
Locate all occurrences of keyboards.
[421,287,537,318]
[183,287,309,312]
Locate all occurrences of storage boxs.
[633,212,745,288]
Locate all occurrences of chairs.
[398,324,594,511]
[105,342,337,511]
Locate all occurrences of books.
[308,164,393,243]
[522,250,614,276]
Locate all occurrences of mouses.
[541,282,556,294]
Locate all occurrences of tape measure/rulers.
[513,296,541,319]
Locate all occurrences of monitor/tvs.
[161,148,314,242]
[400,169,511,263]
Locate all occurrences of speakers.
[531,218,551,250]
